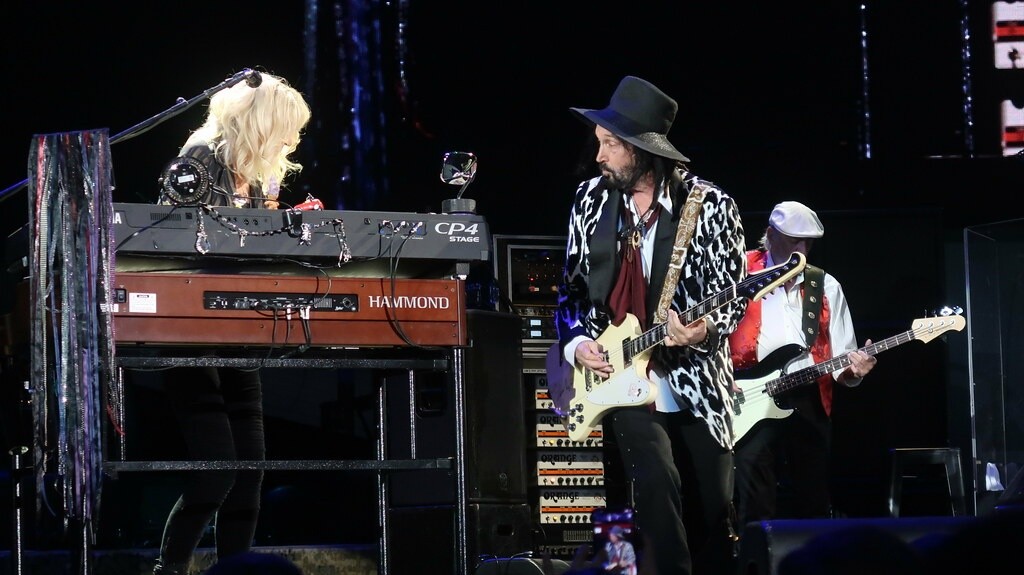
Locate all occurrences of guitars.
[543,249,811,444]
[720,303,970,449]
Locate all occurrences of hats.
[607,526,623,538]
[769,201,824,238]
[569,76,691,162]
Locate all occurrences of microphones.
[246,70,262,88]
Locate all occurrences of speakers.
[387,503,532,575]
[384,309,529,506]
[737,515,978,575]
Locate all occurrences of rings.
[671,334,675,341]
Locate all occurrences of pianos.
[43,200,494,281]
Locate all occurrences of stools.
[889,446,967,517]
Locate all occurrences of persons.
[155,70,312,575]
[731,201,877,575]
[603,525,638,575]
[552,72,751,574]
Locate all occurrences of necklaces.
[628,196,657,251]
